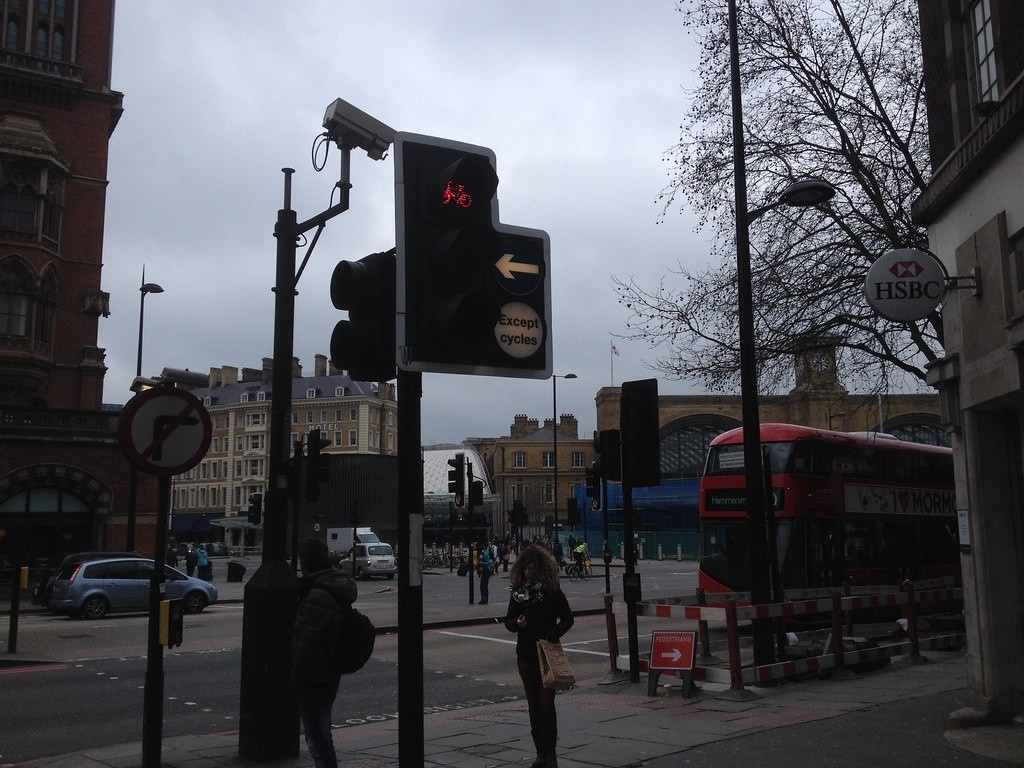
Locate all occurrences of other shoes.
[479,601,488,604]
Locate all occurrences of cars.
[47,558,218,620]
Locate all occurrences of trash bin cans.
[206,561,213,581]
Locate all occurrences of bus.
[696,423,960,630]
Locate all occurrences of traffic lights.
[507,509,514,526]
[522,507,529,525]
[584,461,602,512]
[158,597,182,649]
[248,493,263,525]
[330,246,397,382]
[447,452,466,508]
[394,130,554,381]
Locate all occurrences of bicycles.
[423,549,469,568]
[568,556,592,583]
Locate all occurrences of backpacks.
[310,582,375,676]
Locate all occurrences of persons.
[185,549,197,577]
[196,546,208,580]
[489,534,529,572]
[534,533,564,571]
[167,548,178,568]
[475,543,489,605]
[568,535,587,565]
[504,545,573,768]
[289,536,358,768]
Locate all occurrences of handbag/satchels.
[537,640,575,692]
[477,560,493,578]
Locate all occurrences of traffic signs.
[650,632,695,670]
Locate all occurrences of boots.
[529,702,557,768]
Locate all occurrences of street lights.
[724,179,836,689]
[552,373,578,540]
[123,262,164,553]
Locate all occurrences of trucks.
[326,527,397,580]
